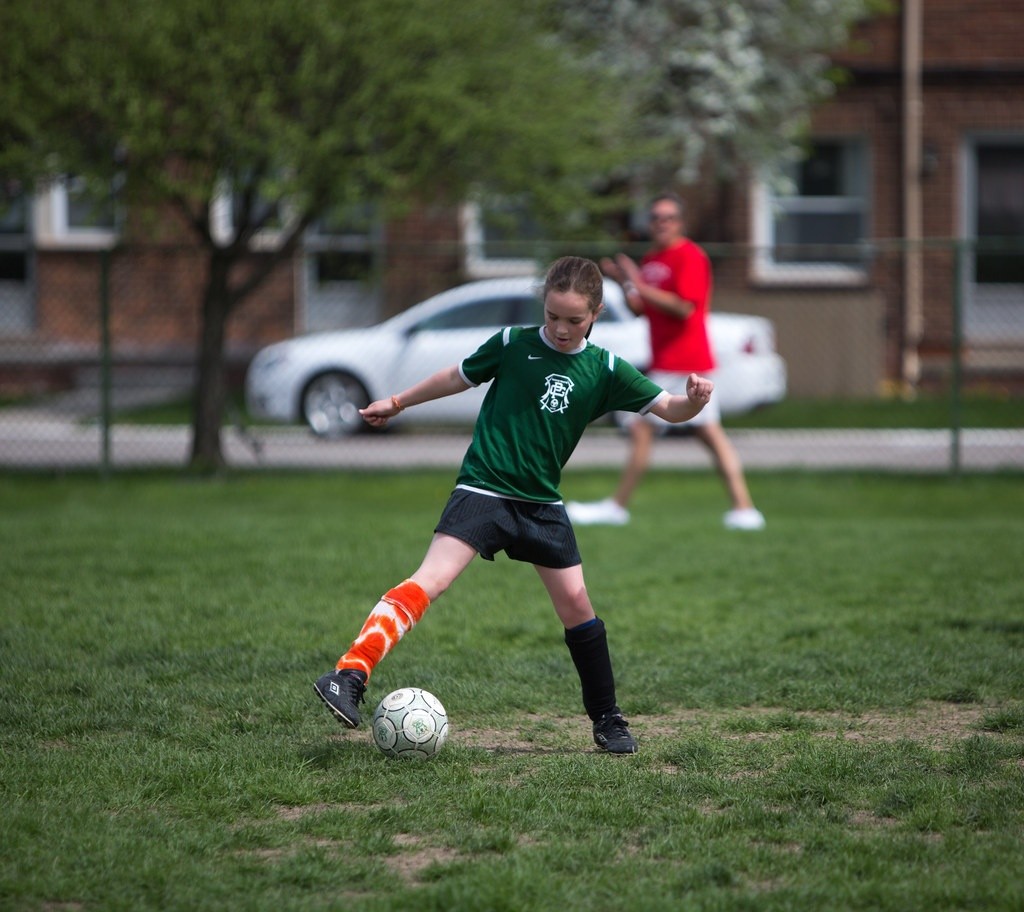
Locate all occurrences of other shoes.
[565,497,630,526]
[725,506,766,533]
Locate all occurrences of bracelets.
[392,397,405,411]
[623,280,635,293]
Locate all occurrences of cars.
[245,272,793,443]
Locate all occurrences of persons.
[314,256,714,754]
[564,192,764,530]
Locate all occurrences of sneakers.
[591,709,638,756]
[313,670,367,729]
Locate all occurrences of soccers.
[370,687,450,761]
